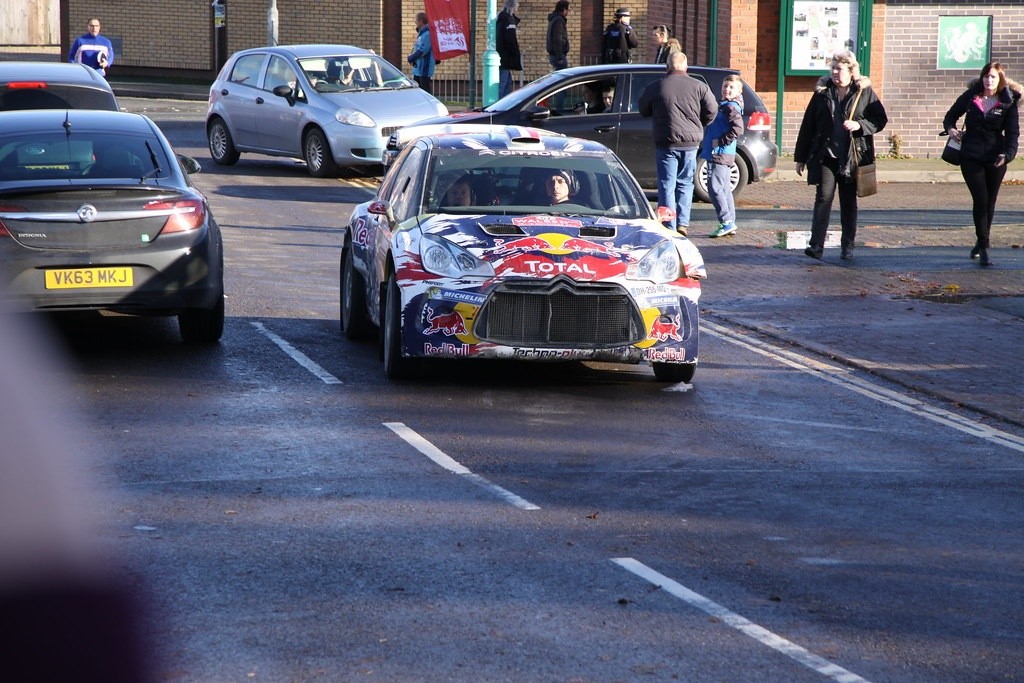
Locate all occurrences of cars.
[0,55,121,112]
[205,44,450,178]
[0,108,224,345]
[340,126,706,382]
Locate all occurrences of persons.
[652,25,682,64]
[547,0,570,110]
[794,51,888,260]
[408,13,436,93]
[544,168,576,204]
[943,61,1023,265]
[68,17,114,77]
[496,0,522,99]
[573,80,615,115]
[638,52,718,234]
[88,137,142,178]
[601,7,639,64]
[330,59,364,89]
[699,74,745,238]
[445,174,477,206]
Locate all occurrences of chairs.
[437,183,472,209]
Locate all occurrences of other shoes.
[804,245,823,259]
[970,242,992,267]
[678,224,688,237]
[839,245,854,259]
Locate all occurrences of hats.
[614,8,630,16]
[544,169,574,188]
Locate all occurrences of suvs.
[381,64,778,204]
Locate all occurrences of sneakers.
[708,220,738,237]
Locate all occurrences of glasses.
[89,24,100,28]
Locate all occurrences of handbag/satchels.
[941,131,963,166]
[857,164,877,197]
